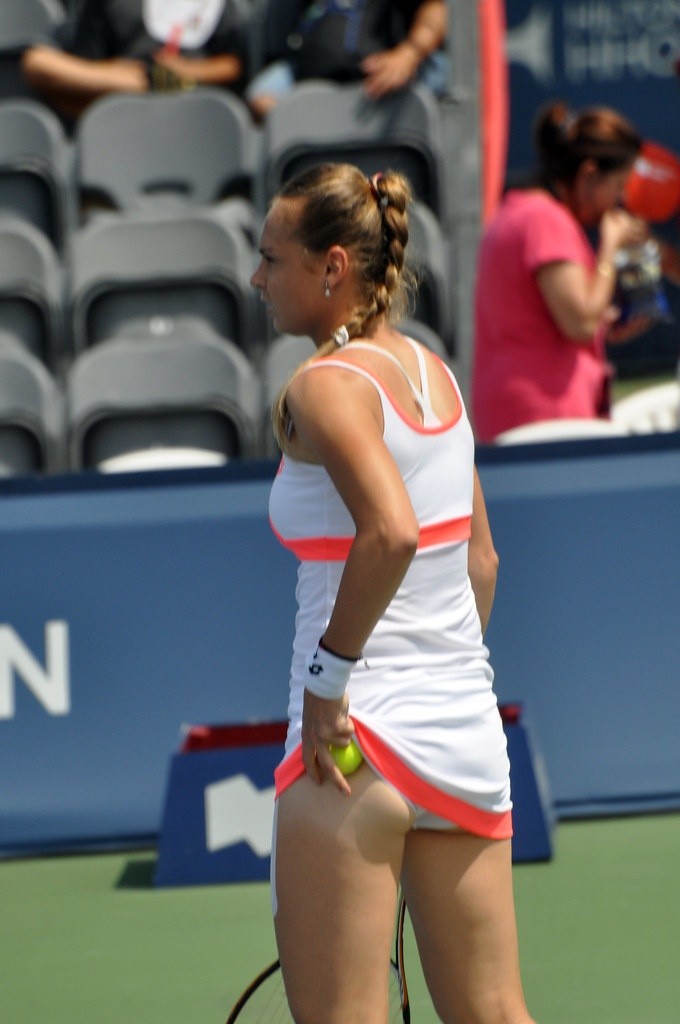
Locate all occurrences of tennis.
[328,737,362,776]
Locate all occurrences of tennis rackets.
[227,887,412,1024]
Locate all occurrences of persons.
[246,162,533,1024]
[246,0,460,116]
[609,241,680,376]
[22,0,255,133]
[470,107,652,446]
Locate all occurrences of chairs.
[389,200,451,348]
[67,334,262,474]
[69,216,259,374]
[0,347,67,476]
[0,100,70,261]
[0,217,60,370]
[72,93,257,253]
[264,317,450,467]
[260,79,447,225]
[0,0,73,144]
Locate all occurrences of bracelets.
[406,38,431,63]
[304,637,365,701]
